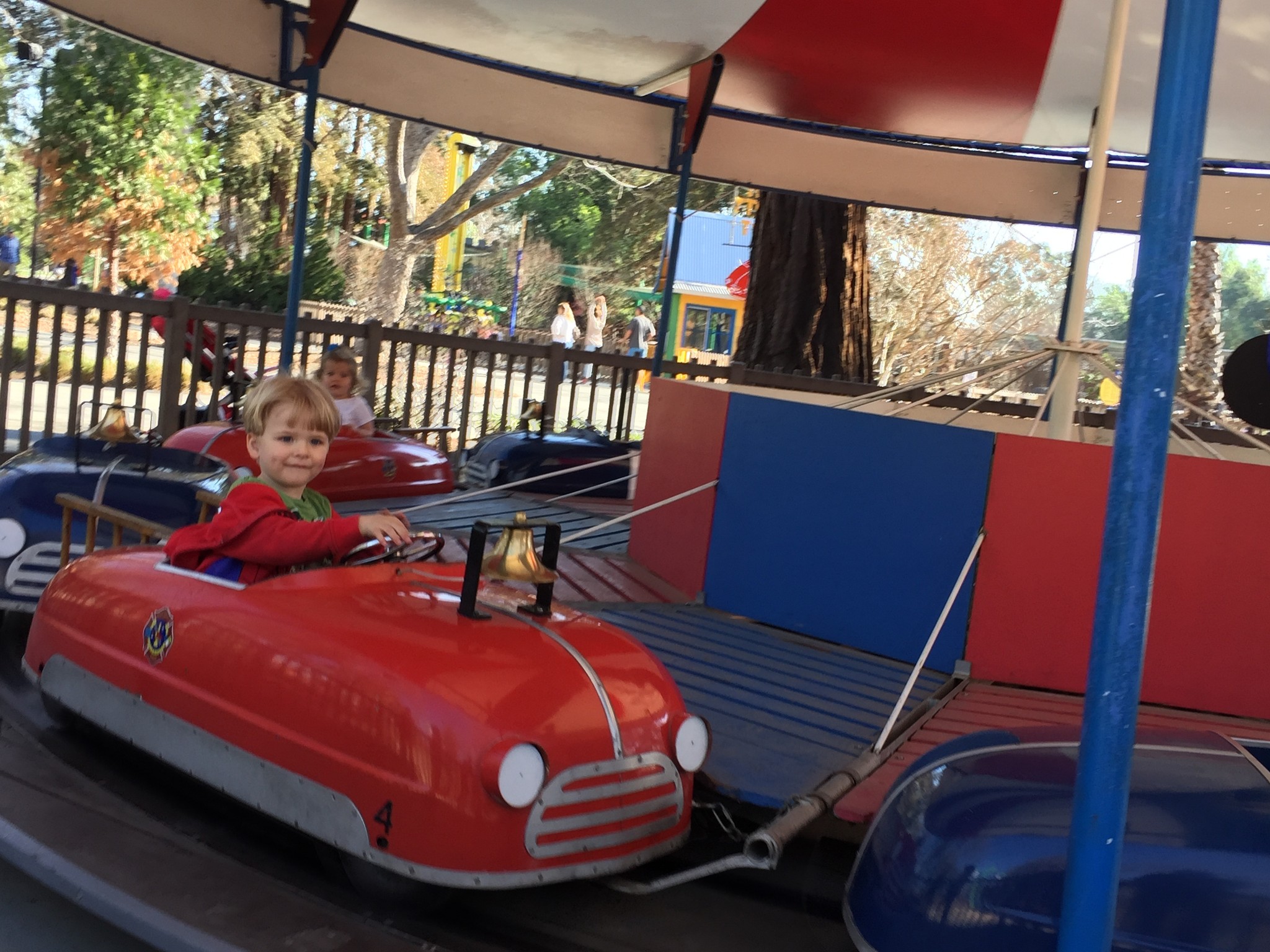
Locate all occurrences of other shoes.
[627,386,631,391]
[578,377,592,383]
[614,383,622,388]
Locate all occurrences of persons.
[0,228,21,275]
[161,374,413,585]
[318,348,375,437]
[62,259,77,286]
[551,296,656,392]
